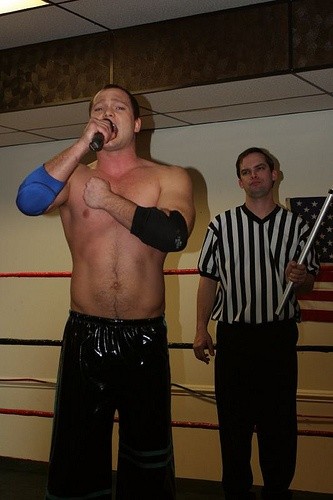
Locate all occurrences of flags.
[282,191,333,351]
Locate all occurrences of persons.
[191,148,321,500]
[16,85,196,500]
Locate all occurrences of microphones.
[89,118,113,152]
